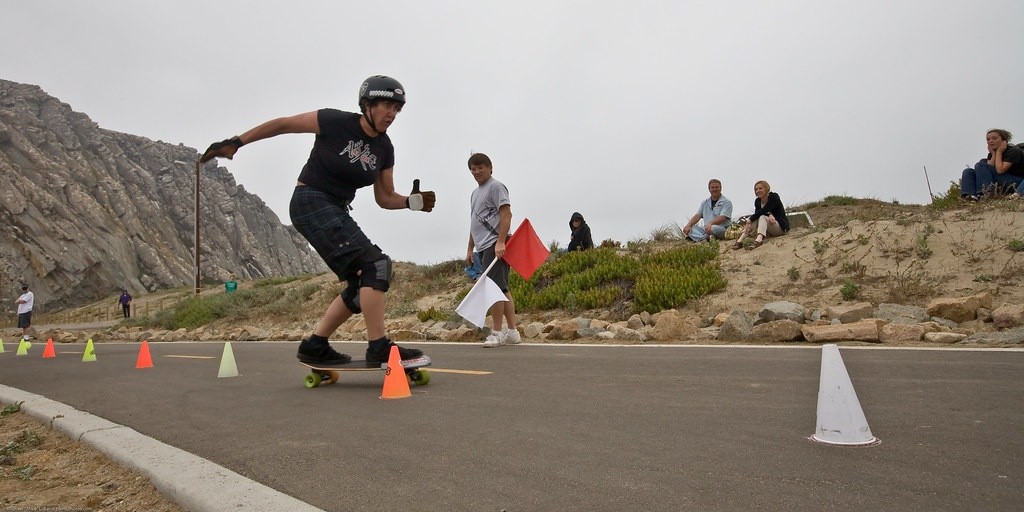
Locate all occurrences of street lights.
[173,160,201,295]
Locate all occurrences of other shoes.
[748,241,762,249]
[731,241,744,249]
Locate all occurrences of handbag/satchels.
[725,221,744,239]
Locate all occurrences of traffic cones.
[15,338,28,355]
[42,338,56,358]
[380,346,413,399]
[134,340,153,369]
[810,344,877,446]
[81,338,97,362]
[218,342,239,378]
[0,339,5,353]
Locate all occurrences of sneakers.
[296,337,353,365]
[363,340,423,366]
[482,331,505,348]
[504,331,521,344]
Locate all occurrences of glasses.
[572,219,579,222]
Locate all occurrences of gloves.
[406,179,436,214]
[200,135,242,163]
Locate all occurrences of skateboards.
[299,355,432,389]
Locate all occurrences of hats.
[22,284,29,290]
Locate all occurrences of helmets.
[358,74,407,107]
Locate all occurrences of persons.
[961,129,1024,201]
[14,284,33,341]
[466,152,522,347]
[224,274,237,294]
[200,75,436,367]
[732,180,791,250]
[683,179,733,243]
[568,212,594,252]
[118,289,132,318]
